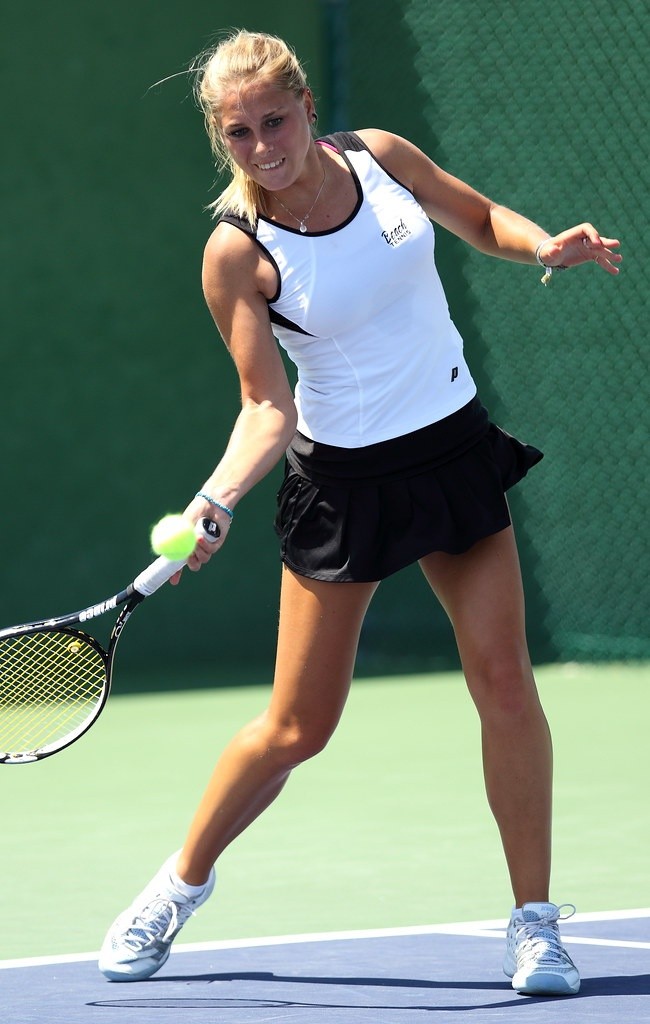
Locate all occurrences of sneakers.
[98,848,217,982]
[500,900,582,995]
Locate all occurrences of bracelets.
[536,237,553,284]
[196,492,233,517]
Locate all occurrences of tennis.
[146,514,204,569]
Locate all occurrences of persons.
[100,31,621,996]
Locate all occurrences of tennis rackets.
[1,508,225,768]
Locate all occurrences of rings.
[583,238,589,248]
[595,256,599,265]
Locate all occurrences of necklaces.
[272,168,327,233]
